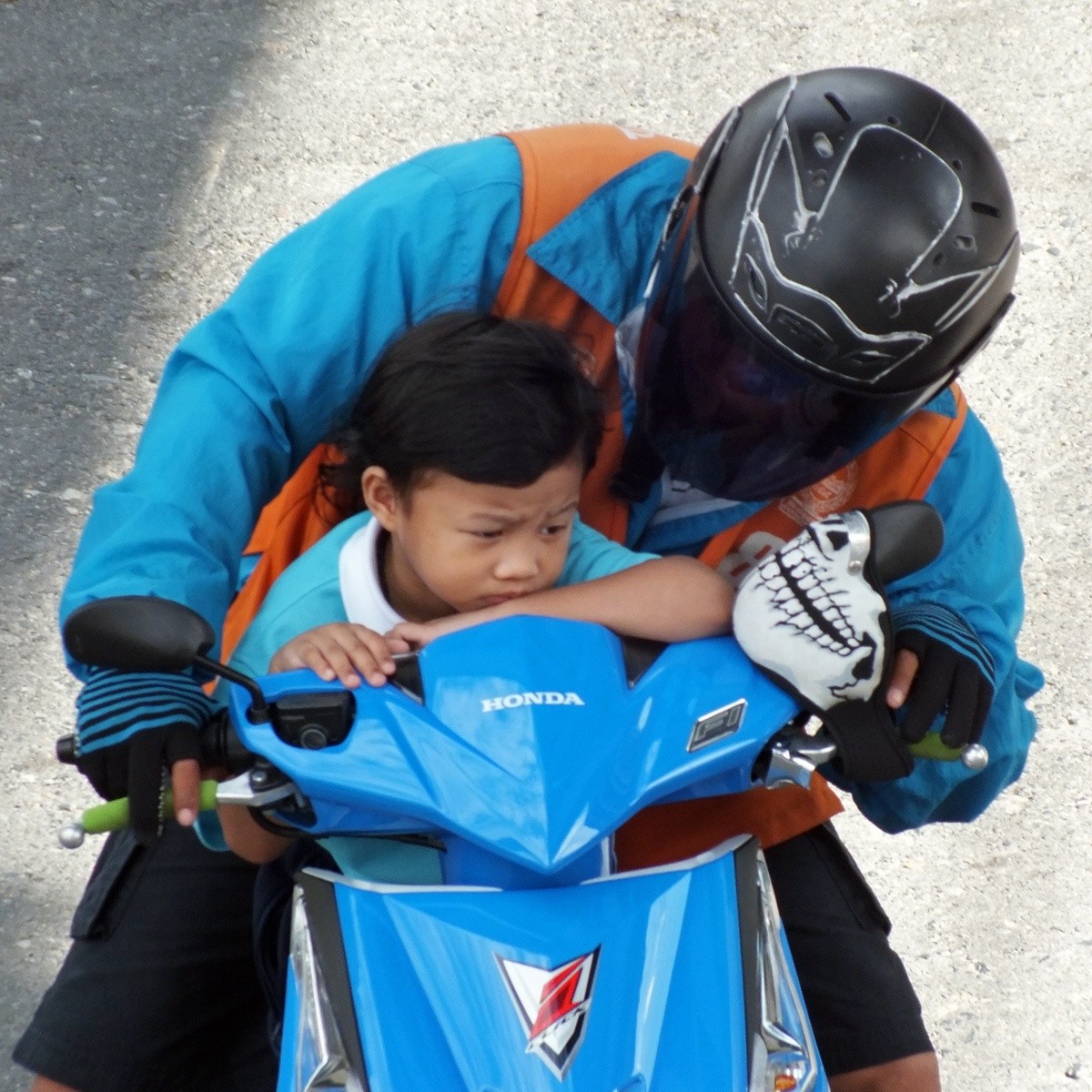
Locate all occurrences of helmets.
[632,66,1024,503]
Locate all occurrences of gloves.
[76,663,222,838]
[895,630,992,746]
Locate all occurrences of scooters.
[47,496,995,1092]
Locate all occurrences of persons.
[214,312,732,870]
[12,67,1044,1091]
